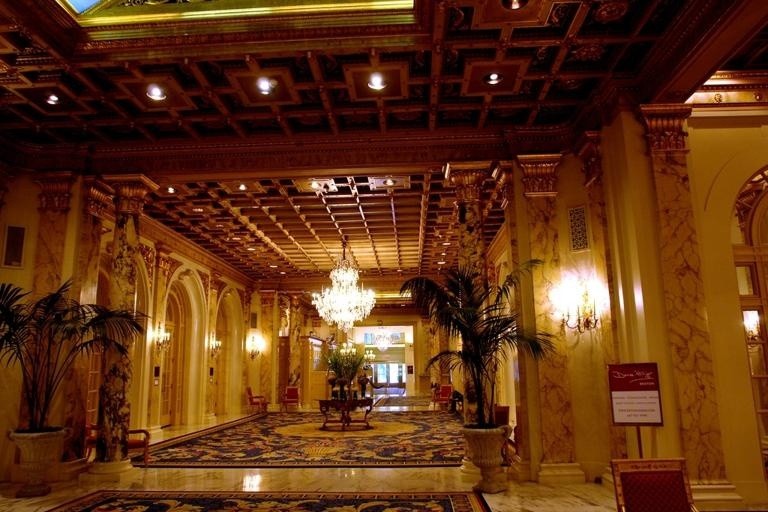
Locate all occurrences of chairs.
[609,457,701,512]
[433,384,453,410]
[245,385,267,414]
[279,386,300,413]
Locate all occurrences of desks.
[318,398,373,429]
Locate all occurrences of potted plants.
[396,256,560,493]
[0,275,152,497]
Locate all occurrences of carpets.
[130,410,512,467]
[46,489,492,512]
[373,395,430,406]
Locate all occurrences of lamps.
[311,242,377,334]
